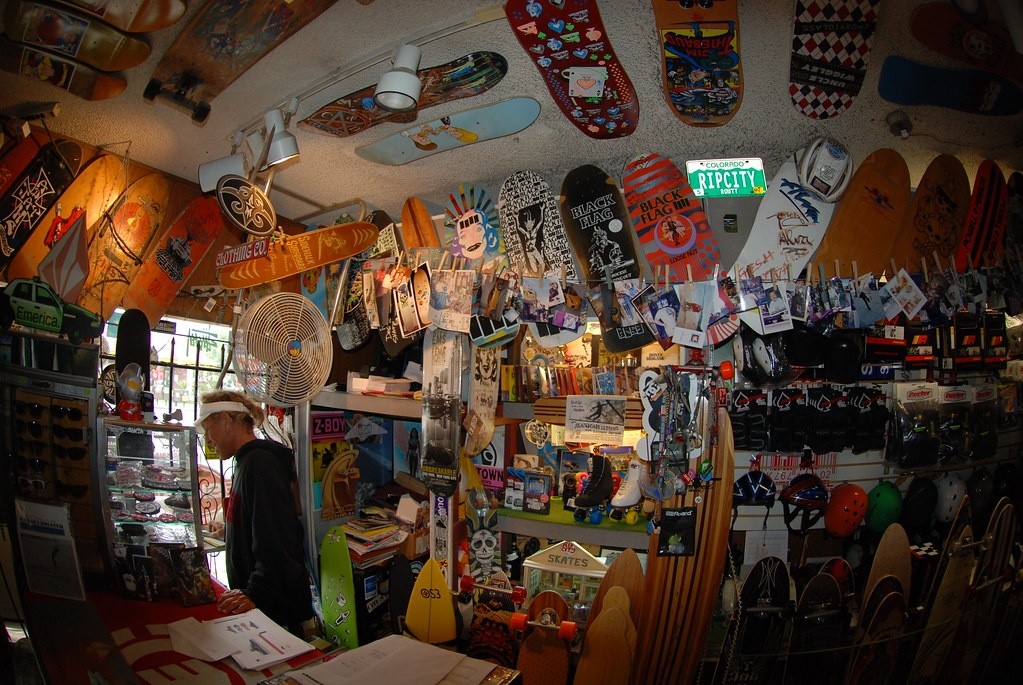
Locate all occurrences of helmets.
[730,461,1023,540]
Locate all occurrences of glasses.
[1,397,91,505]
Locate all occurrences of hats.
[193,401,251,434]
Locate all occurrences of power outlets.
[192,285,240,297]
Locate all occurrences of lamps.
[197,132,248,194]
[375,43,422,112]
[263,97,299,166]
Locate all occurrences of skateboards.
[0,0,1021,422]
[320,490,1023,685]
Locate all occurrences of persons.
[638,302,657,333]
[435,277,449,294]
[655,299,676,336]
[769,291,786,314]
[744,289,757,307]
[828,284,837,305]
[856,286,871,310]
[193,390,316,628]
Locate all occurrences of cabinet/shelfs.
[97,415,209,576]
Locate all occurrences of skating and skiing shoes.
[573,454,613,524]
[609,460,649,525]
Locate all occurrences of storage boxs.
[504,452,553,516]
[401,527,432,561]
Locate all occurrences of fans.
[235,291,333,408]
